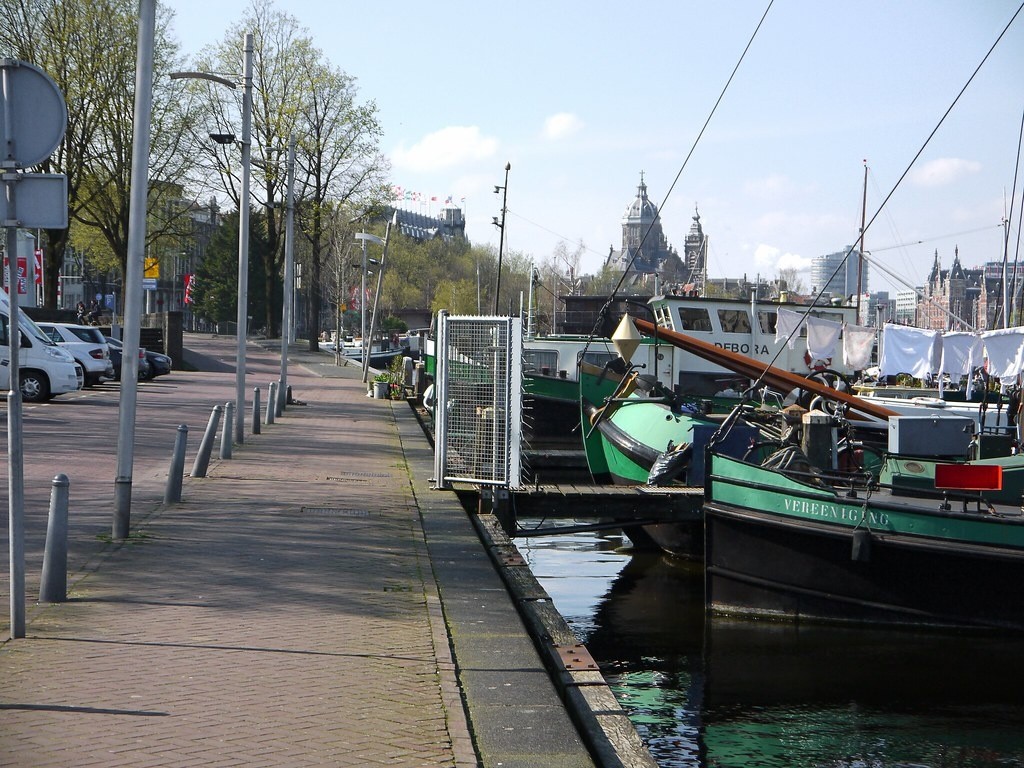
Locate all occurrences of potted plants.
[374,355,411,401]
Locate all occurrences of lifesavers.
[910,396,946,409]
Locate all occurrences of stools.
[403,386,414,399]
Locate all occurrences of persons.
[78,301,86,325]
[87,300,103,326]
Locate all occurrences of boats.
[692,0,1024,642]
[317,0,1024,576]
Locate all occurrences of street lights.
[168,33,256,445]
[489,162,511,316]
[348,216,368,372]
[263,132,296,412]
[354,219,394,382]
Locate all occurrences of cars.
[47,333,149,381]
[138,350,173,381]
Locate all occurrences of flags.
[185,273,196,304]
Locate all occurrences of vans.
[0,285,86,403]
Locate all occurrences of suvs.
[5,321,116,390]
